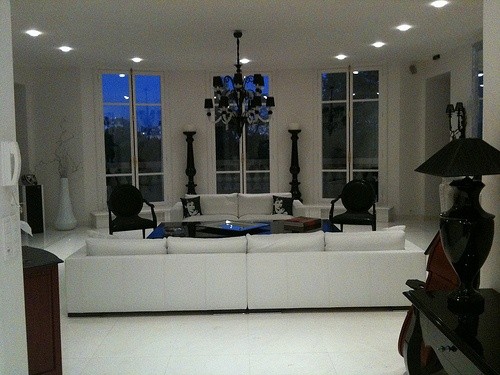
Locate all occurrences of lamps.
[446,102,467,141]
[414,137,500,335]
[204,30,277,138]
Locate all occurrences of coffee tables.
[144,217,343,237]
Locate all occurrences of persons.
[274,198,288,214]
[185,199,200,217]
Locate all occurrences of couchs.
[63,230,425,317]
[170,192,306,225]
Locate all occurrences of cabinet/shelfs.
[22,247,64,374]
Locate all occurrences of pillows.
[179,196,202,218]
[271,195,294,216]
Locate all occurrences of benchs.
[305,205,393,223]
[90,209,170,229]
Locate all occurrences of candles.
[288,122,301,131]
[184,125,195,130]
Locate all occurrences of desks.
[398,286,500,375]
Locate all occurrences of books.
[284,216,321,227]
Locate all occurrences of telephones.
[0,140,22,187]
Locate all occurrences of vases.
[53,177,76,230]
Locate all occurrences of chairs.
[329,179,379,231]
[106,184,157,238]
